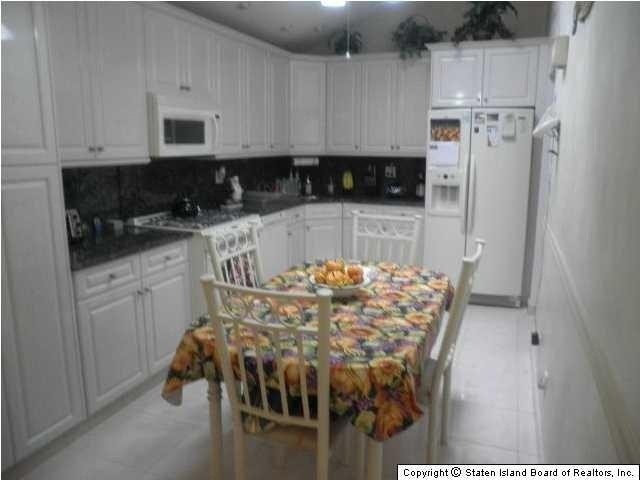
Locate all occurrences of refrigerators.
[423,107,534,308]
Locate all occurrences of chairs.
[352,209,422,263]
[205,219,264,302]
[416,239,484,464]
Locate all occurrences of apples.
[314,259,362,286]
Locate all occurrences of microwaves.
[147,108,221,159]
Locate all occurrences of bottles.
[416,172,424,200]
[274,168,312,197]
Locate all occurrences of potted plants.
[454,1,517,40]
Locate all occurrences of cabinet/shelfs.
[43,2,149,167]
[149,1,215,96]
[74,240,189,416]
[290,59,324,155]
[258,206,303,280]
[425,38,547,107]
[2,164,86,480]
[304,203,342,262]
[267,54,290,156]
[361,60,430,157]
[216,33,267,159]
[2,1,50,164]
[200,274,363,480]
[343,203,424,264]
[324,60,362,156]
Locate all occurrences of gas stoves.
[130,207,260,235]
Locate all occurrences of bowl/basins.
[309,272,369,299]
